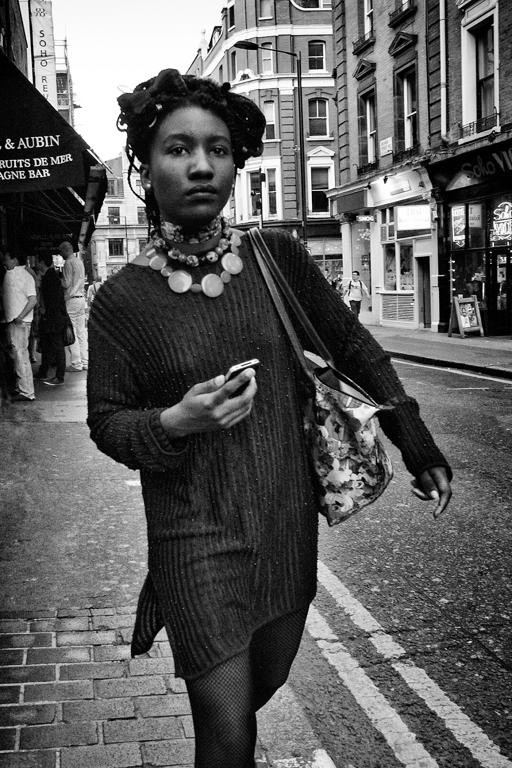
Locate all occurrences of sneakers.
[42,376,65,386]
[7,390,35,401]
[33,373,50,380]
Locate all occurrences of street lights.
[231,40,308,253]
[105,214,130,264]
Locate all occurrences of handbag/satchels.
[303,366,396,527]
[36,313,74,353]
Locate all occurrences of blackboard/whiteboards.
[454,295,483,331]
[449,297,458,329]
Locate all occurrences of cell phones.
[225,359,260,399]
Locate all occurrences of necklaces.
[145,216,245,298]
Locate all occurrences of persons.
[0,241,105,403]
[342,271,370,318]
[85,66,453,767]
